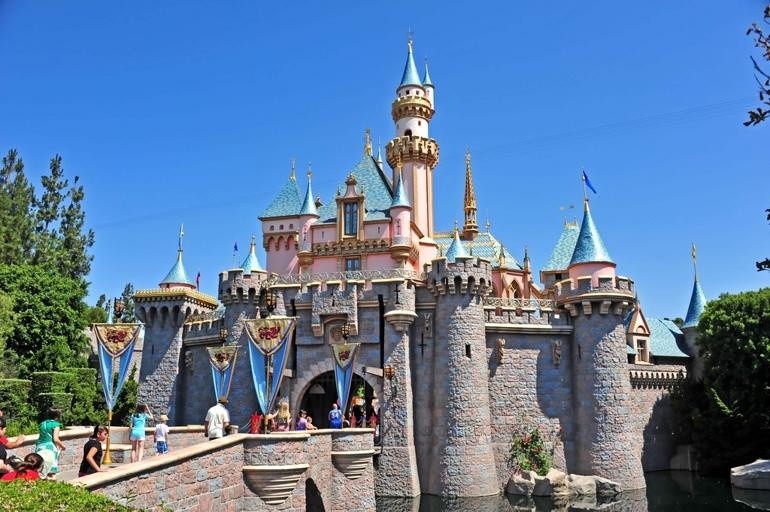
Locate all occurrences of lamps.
[384,363,397,397]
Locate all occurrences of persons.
[223,425,235,436]
[35,408,65,481]
[154,414,171,455]
[275,401,293,433]
[129,402,153,463]
[328,402,381,445]
[0,408,44,481]
[306,416,317,430]
[204,396,231,441]
[294,410,317,430]
[79,424,109,478]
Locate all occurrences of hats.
[159,415,169,422]
[219,397,227,403]
